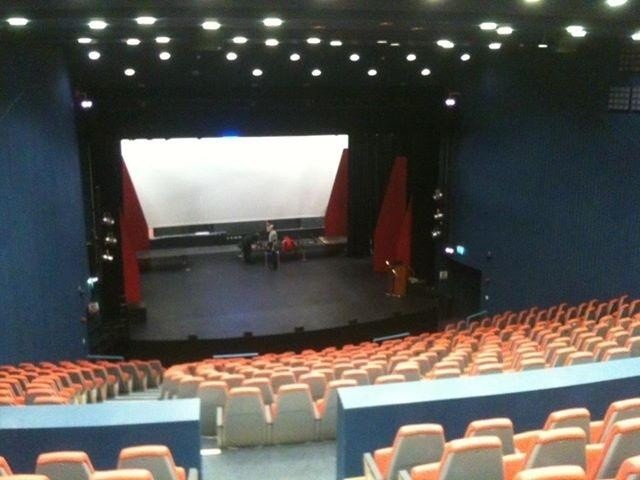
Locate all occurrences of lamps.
[93,203,119,266]
[429,185,448,240]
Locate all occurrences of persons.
[266,225,279,250]
[265,220,275,239]
[264,242,276,270]
[281,235,298,252]
[238,232,253,263]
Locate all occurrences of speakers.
[132,307,147,320]
[349,318,358,324]
[392,310,402,318]
[295,326,304,332]
[189,333,197,340]
[243,331,252,337]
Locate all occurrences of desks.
[251,233,349,261]
